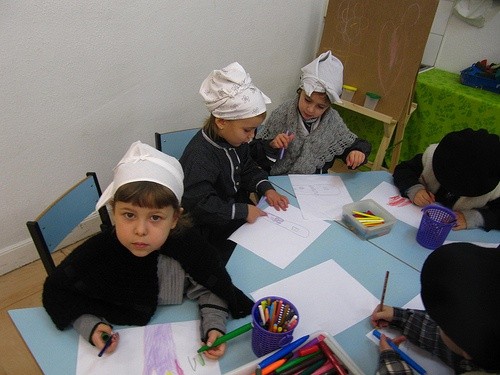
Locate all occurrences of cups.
[340,85,358,102]
[363,93,381,111]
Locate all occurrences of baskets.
[461,67,500,93]
[416,206,458,250]
[251,296,300,357]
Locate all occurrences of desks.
[401,66,500,161]
[9,171,500,375]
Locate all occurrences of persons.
[41,141,256,359]
[255,50,372,175]
[178,63,289,265]
[393,128,500,232]
[372,243,500,375]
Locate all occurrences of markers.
[256,335,350,375]
[198,322,254,353]
[373,330,427,375]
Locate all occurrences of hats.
[95,140,184,212]
[422,242,500,374]
[297,50,344,103]
[433,128,500,196]
[199,62,272,120]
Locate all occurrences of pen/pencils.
[422,175,431,199]
[254,297,298,333]
[378,271,390,327]
[279,129,289,160]
[98,336,112,357]
[351,210,385,228]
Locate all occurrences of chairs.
[155,128,200,161]
[27,170,111,271]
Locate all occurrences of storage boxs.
[224,331,362,375]
[344,199,394,239]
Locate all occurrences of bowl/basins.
[343,199,397,240]
